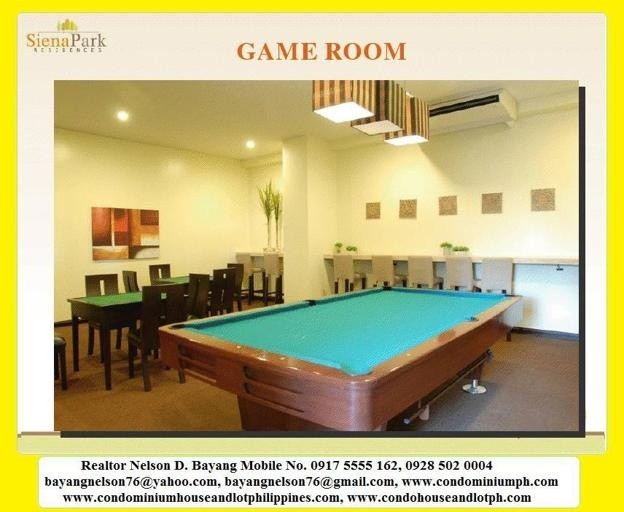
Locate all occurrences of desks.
[162,287,523,431]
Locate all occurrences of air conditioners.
[429,89,517,136]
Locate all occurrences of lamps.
[312,80,430,147]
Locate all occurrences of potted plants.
[335,242,358,256]
[440,242,469,257]
[255,176,280,252]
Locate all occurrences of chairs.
[333,254,515,342]
[54,252,284,391]
